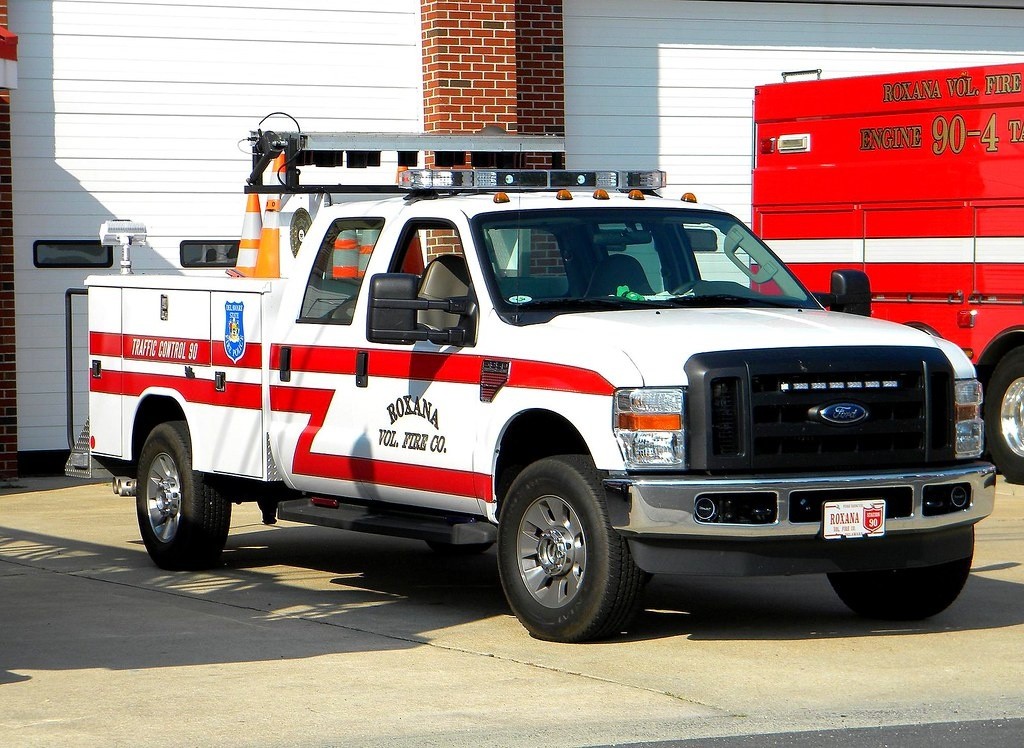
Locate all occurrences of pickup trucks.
[65,111,996,645]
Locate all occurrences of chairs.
[416,254,470,328]
[582,253,658,296]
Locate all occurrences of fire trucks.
[749,63,1024,484]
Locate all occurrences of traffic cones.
[358,230,380,279]
[236,150,286,278]
[402,230,425,276]
[333,229,359,278]
